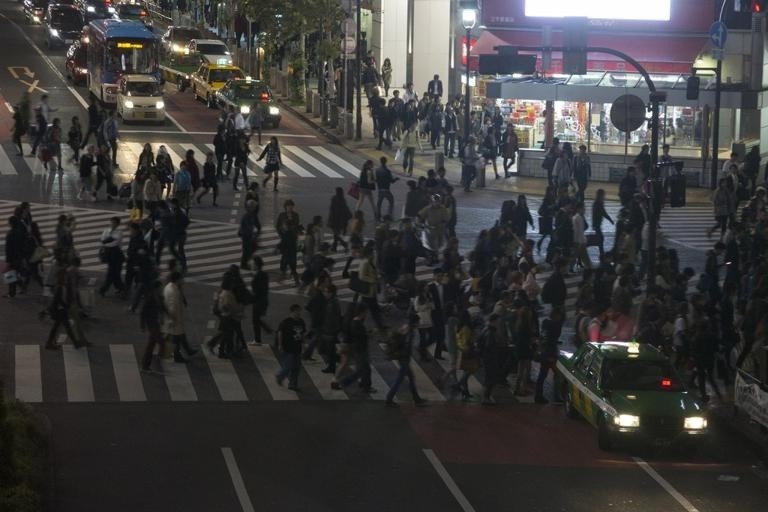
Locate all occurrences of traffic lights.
[753,0,766,13]
[686,76,699,100]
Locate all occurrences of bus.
[82,15,164,107]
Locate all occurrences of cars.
[552,340,712,449]
[189,63,248,109]
[216,77,283,130]
[22,1,234,92]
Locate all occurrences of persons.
[1,1,766,409]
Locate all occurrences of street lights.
[460,0,481,139]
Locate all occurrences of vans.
[116,71,166,124]
